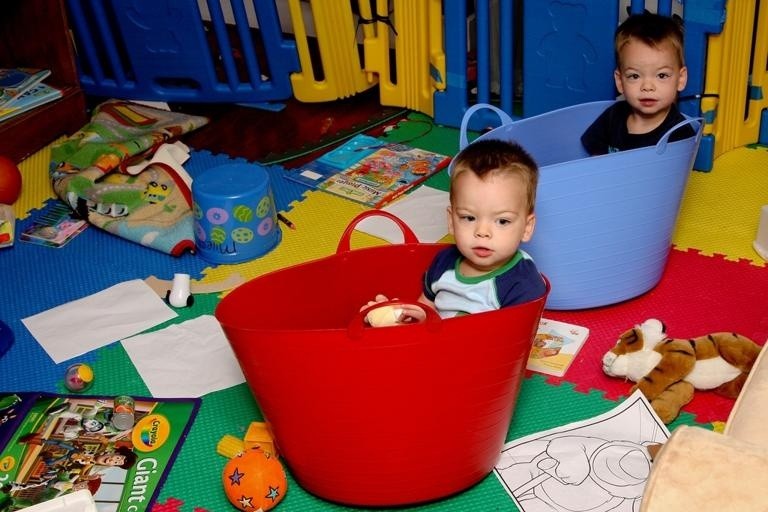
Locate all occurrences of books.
[525,318,590,377]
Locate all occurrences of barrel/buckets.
[190,163,283,264]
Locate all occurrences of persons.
[0,399,138,512]
[357,137,547,319]
[579,13,697,157]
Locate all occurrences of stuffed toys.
[600,318,763,424]
